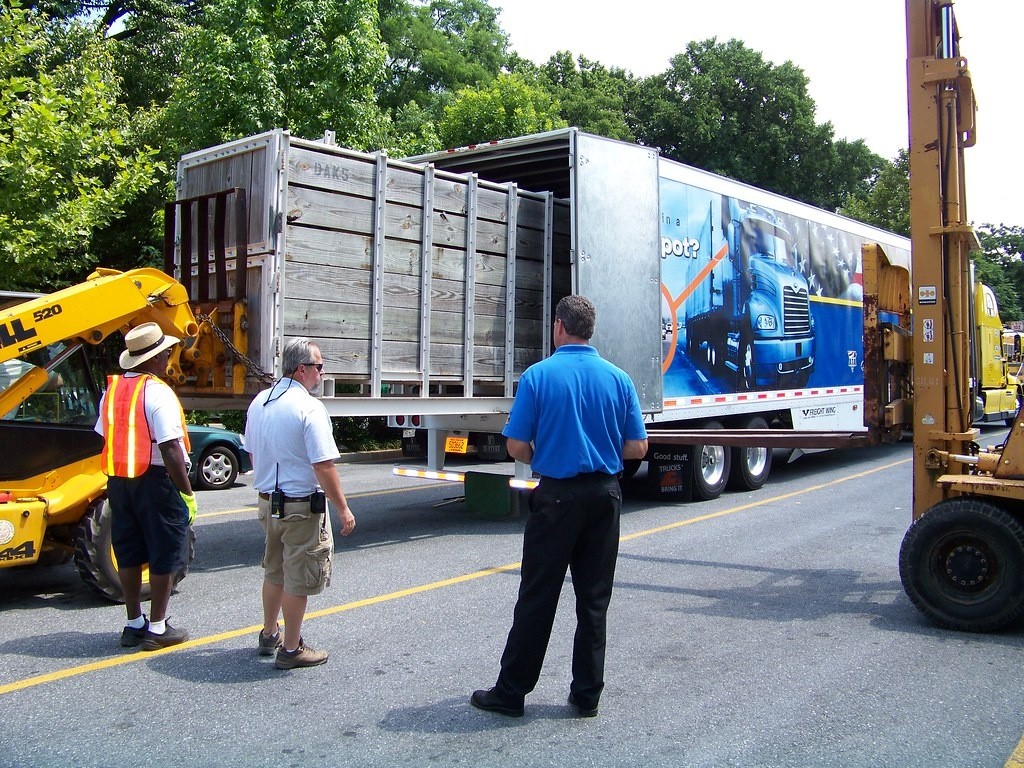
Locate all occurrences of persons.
[471,296,649,718]
[93,321,199,651]
[0,354,65,421]
[242,338,355,670]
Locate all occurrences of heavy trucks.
[403,124,1024,503]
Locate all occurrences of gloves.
[179,490,197,526]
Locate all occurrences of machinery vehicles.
[898,2,1024,635]
[0,265,254,605]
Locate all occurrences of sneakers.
[120,614,149,647]
[142,616,189,651]
[274,636,329,670]
[258,622,285,656]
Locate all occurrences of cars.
[54,386,253,493]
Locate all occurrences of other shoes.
[471,687,524,717]
[569,692,598,717]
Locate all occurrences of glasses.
[295,362,324,372]
[164,347,173,356]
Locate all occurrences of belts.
[259,491,312,503]
[541,471,617,480]
[144,464,170,479]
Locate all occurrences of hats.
[118,322,181,370]
